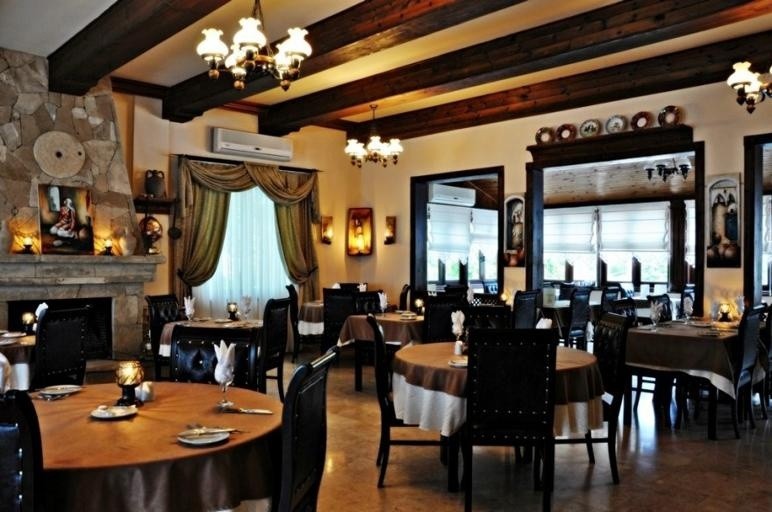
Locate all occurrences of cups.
[455,340,463,356]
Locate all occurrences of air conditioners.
[427,184,476,208]
[212,126,294,162]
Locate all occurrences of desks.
[161,319,263,387]
[301,299,391,359]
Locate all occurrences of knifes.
[400,318,415,320]
[36,386,88,391]
[709,328,735,333]
[181,429,238,437]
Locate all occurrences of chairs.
[2,388,48,511]
[450,325,556,511]
[422,280,695,340]
[366,314,459,487]
[275,350,339,512]
[28,303,93,388]
[168,321,259,392]
[243,297,291,404]
[593,309,627,488]
[141,292,179,381]
[673,305,772,436]
[286,283,324,364]
[613,292,695,424]
[323,281,411,365]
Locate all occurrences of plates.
[180,426,231,447]
[215,319,232,323]
[41,384,82,395]
[0,340,16,345]
[89,404,138,420]
[448,356,468,367]
[402,313,416,317]
[2,332,27,338]
[685,321,715,327]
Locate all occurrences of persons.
[49,197,81,238]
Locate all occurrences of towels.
[34,301,50,331]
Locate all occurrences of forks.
[220,405,275,415]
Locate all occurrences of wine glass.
[214,360,237,408]
[682,306,694,321]
[649,304,662,328]
[185,307,195,323]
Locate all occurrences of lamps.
[195,0,314,92]
[341,103,403,168]
[114,360,146,407]
[724,60,771,112]
[643,155,690,182]
